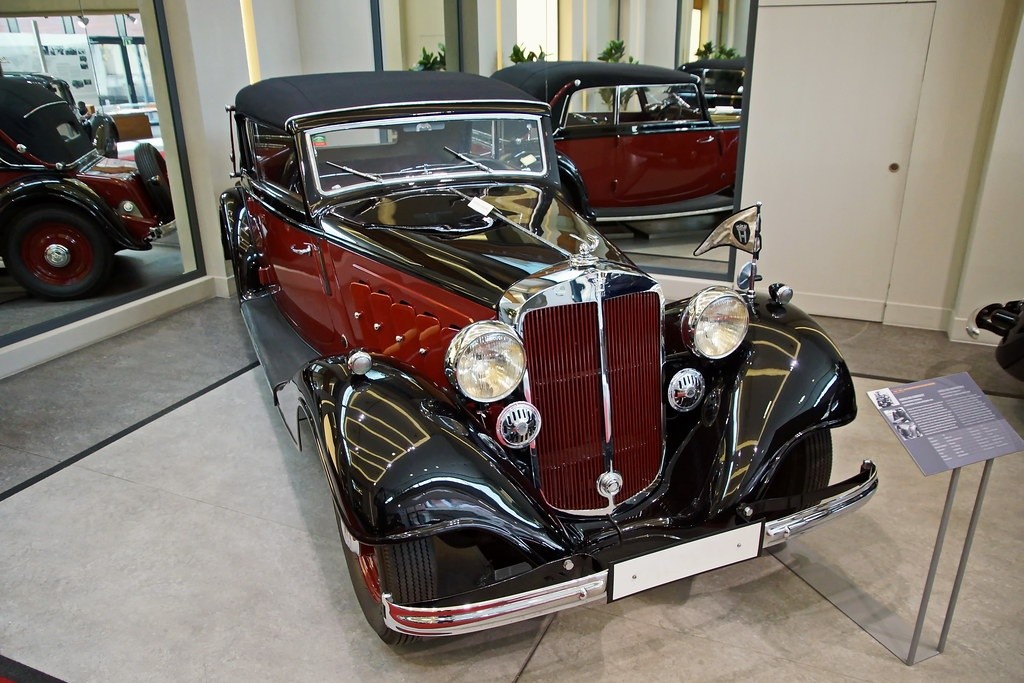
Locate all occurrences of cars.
[666,60,746,116]
[470,59,744,222]
[0,71,177,302]
[217,72,883,650]
[24,72,121,161]
